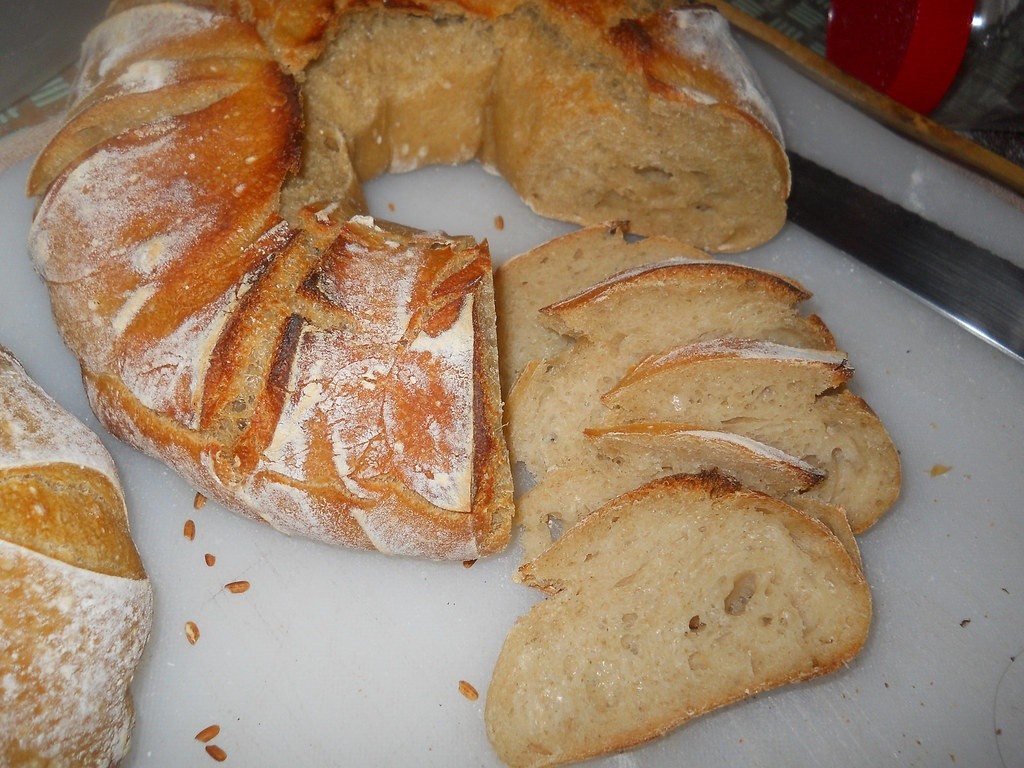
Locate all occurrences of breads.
[26,0,905,767]
[1,346,153,767]
